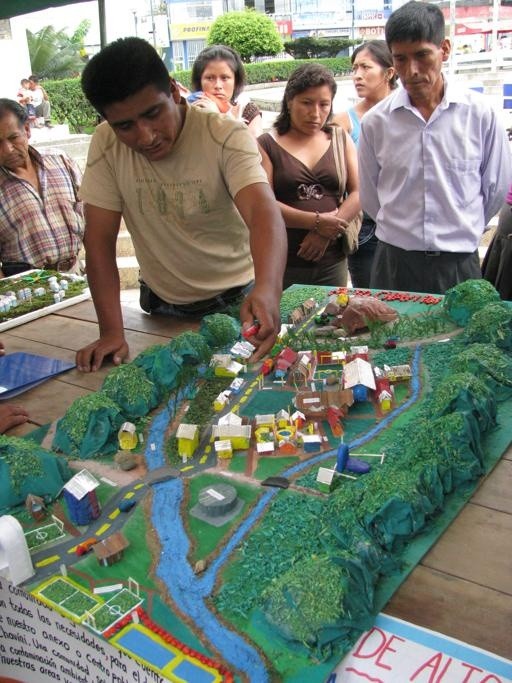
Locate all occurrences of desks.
[0,295,512,680]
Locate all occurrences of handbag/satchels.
[343,211,364,250]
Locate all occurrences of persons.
[18,79,36,122]
[188,43,265,141]
[256,60,364,289]
[328,38,400,289]
[28,76,54,128]
[73,35,289,371]
[0,98,85,280]
[1,402,28,435]
[357,3,512,298]
[176,80,234,123]
[481,171,512,302]
[0,341,7,355]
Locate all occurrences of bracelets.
[310,210,322,234]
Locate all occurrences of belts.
[154,287,247,316]
[409,249,448,257]
[43,257,76,273]
